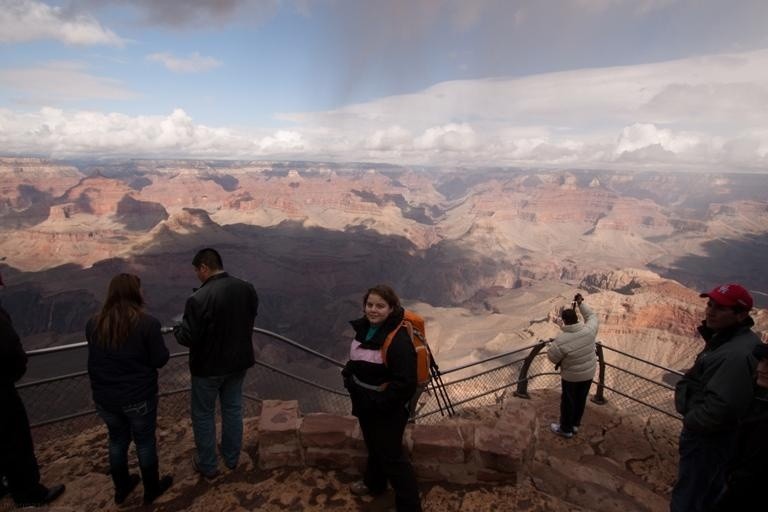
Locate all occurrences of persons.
[0,276,66,507]
[176,246,259,479]
[340,285,421,511]
[712,342,768,511]
[548,292,599,440]
[669,282,768,511]
[84,273,175,504]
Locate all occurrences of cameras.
[574,292,582,301]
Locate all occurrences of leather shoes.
[35,484,65,507]
[146,476,173,503]
[115,474,141,503]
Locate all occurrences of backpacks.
[381,310,433,385]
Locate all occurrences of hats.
[752,342,768,360]
[700,284,753,312]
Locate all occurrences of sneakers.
[350,480,392,496]
[192,454,218,484]
[551,423,573,438]
